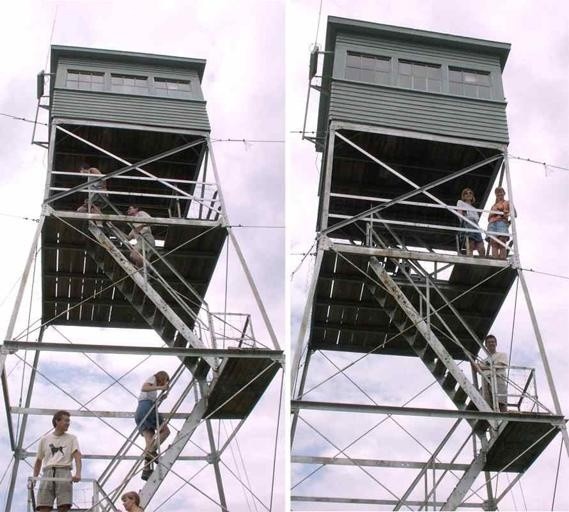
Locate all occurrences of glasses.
[465,192,472,194]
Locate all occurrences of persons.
[135,371,169,480]
[34,411,82,512]
[127,204,155,267]
[121,492,144,512]
[457,188,485,256]
[475,335,509,412]
[485,188,516,259]
[77,163,107,230]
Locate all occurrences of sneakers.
[141,451,158,481]
